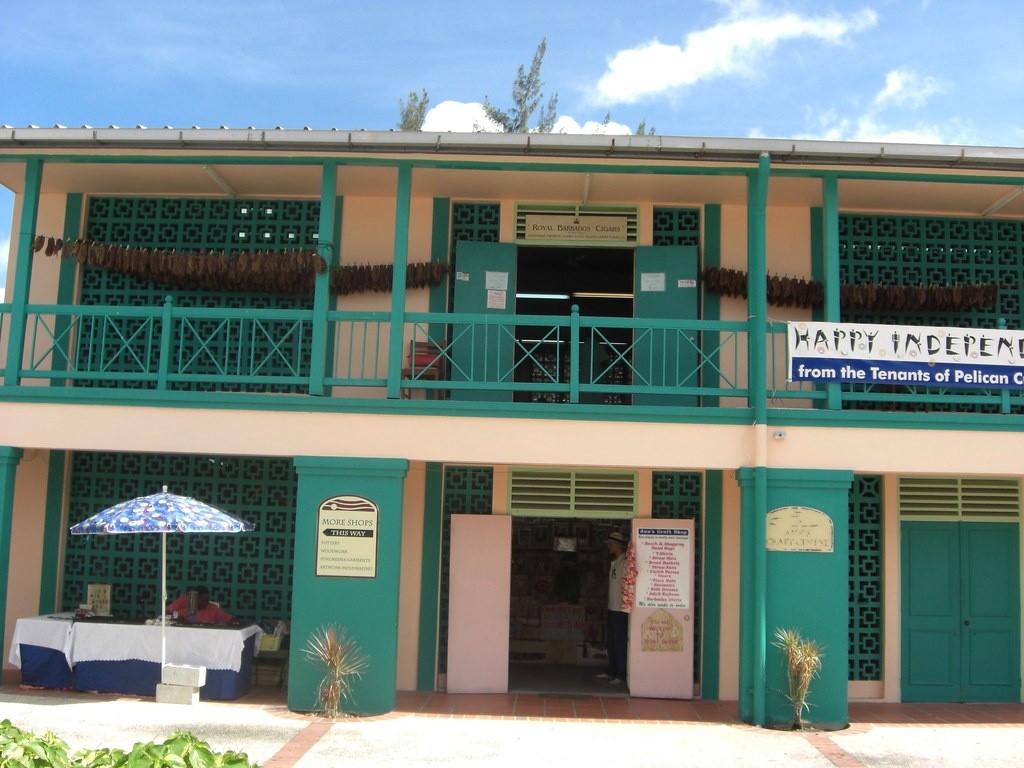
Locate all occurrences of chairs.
[400,340,446,400]
[249,618,290,688]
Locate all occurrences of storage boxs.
[259,634,283,652]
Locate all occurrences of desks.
[8,612,263,701]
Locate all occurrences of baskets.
[260,633,284,651]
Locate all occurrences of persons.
[165,586,240,626]
[595,532,629,685]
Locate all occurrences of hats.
[602,532,627,549]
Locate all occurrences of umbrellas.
[69,485,256,683]
